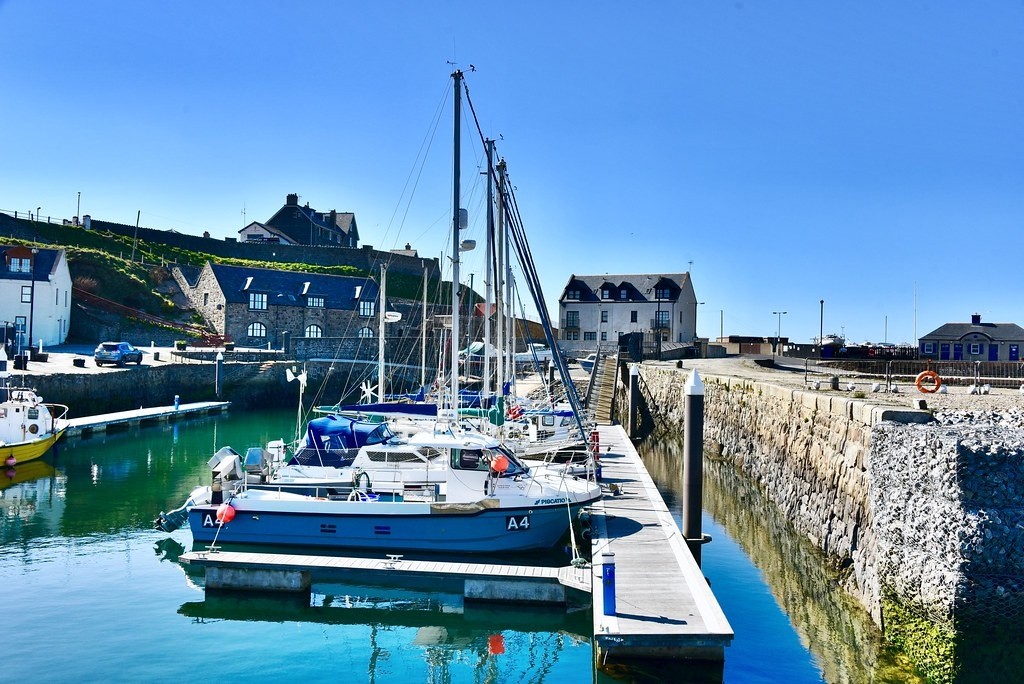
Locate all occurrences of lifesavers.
[29,424,38,434]
[916,371,941,393]
[509,406,523,419]
[869,349,874,355]
[928,358,932,362]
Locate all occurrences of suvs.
[94,341,143,368]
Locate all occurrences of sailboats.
[240,131,614,479]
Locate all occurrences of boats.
[574,352,604,373]
[154,60,604,564]
[0,318,72,470]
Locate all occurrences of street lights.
[656,276,662,348]
[687,302,706,340]
[272,252,276,269]
[77,191,81,226]
[29,249,40,346]
[36,206,42,222]
[819,300,824,360]
[275,293,284,348]
[773,311,787,356]
